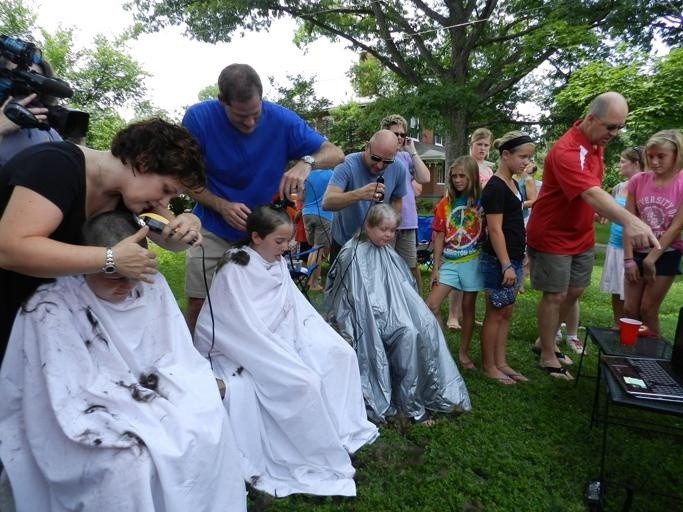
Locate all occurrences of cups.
[617,317,642,347]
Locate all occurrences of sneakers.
[447,320,460,329]
[566,338,586,355]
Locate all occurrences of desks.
[590,357,683,512]
[573,326,673,427]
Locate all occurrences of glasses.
[395,133,405,137]
[633,147,641,161]
[607,125,624,130]
[370,144,395,164]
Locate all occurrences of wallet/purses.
[489,288,515,309]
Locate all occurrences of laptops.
[600,308,683,403]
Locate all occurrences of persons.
[527,92,682,385]
[381,114,430,300]
[322,129,406,268]
[0,118,203,349]
[296,168,337,292]
[427,128,543,384]
[326,202,473,429]
[0,56,63,165]
[182,62,344,344]
[195,193,378,500]
[2,208,248,510]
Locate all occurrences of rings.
[191,230,195,231]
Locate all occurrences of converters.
[587,480,600,506]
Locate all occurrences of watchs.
[103,248,116,274]
[300,155,316,170]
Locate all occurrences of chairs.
[416,215,435,270]
[282,243,324,303]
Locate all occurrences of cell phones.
[403,138,410,145]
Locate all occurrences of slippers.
[461,360,475,368]
[409,409,431,427]
[491,372,528,385]
[532,346,575,381]
